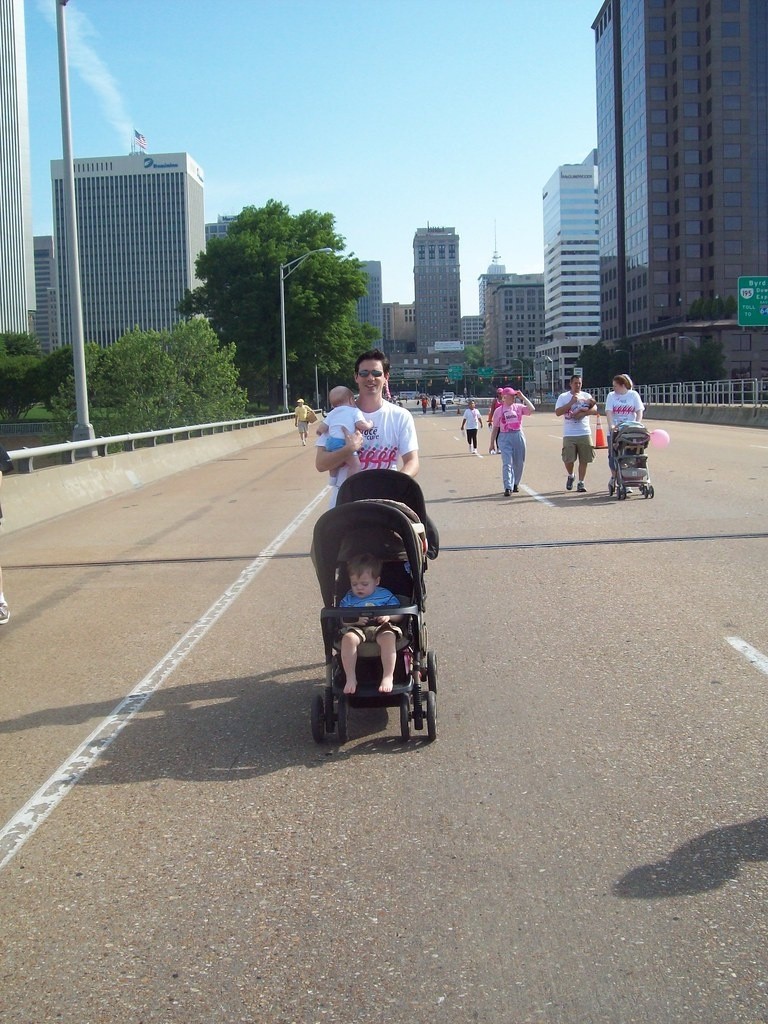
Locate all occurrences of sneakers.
[566,474,576,490]
[625,486,633,493]
[576,482,587,492]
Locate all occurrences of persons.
[0,444,14,624]
[389,395,403,407]
[339,553,402,694]
[316,349,418,508]
[421,395,446,413]
[316,386,373,486]
[461,401,483,453]
[489,387,534,495]
[488,388,504,454]
[566,398,596,419]
[605,375,645,493]
[295,399,314,446]
[554,375,598,492]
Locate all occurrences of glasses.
[358,370,384,377]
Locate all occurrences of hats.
[502,387,517,395]
[497,388,503,393]
[296,398,304,403]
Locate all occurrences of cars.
[440,395,455,404]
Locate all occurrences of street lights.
[513,359,523,393]
[279,247,332,413]
[616,350,631,378]
[541,355,554,397]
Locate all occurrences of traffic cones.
[457,409,461,414]
[594,414,608,449]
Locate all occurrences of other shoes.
[469,444,473,452]
[504,489,511,496]
[497,449,501,454]
[473,448,477,453]
[302,441,306,446]
[513,484,519,492]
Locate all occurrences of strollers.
[310,468,439,744]
[608,421,654,500]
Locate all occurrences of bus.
[399,391,419,399]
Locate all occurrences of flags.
[134,130,146,149]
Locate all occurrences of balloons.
[651,429,669,448]
